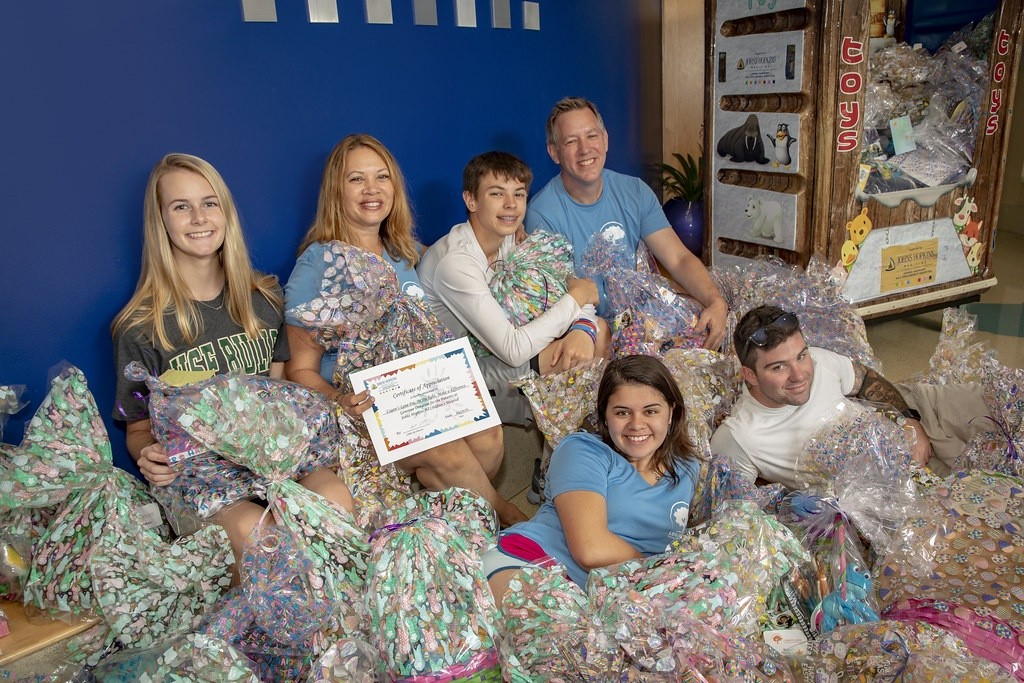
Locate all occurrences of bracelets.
[570,318,597,344]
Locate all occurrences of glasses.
[741,312,800,368]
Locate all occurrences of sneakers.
[528,457,545,506]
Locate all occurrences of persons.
[281,131,531,530]
[524,96,730,351]
[109,152,352,590]
[708,304,954,489]
[412,152,612,502]
[481,354,710,634]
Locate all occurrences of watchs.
[901,408,922,422]
